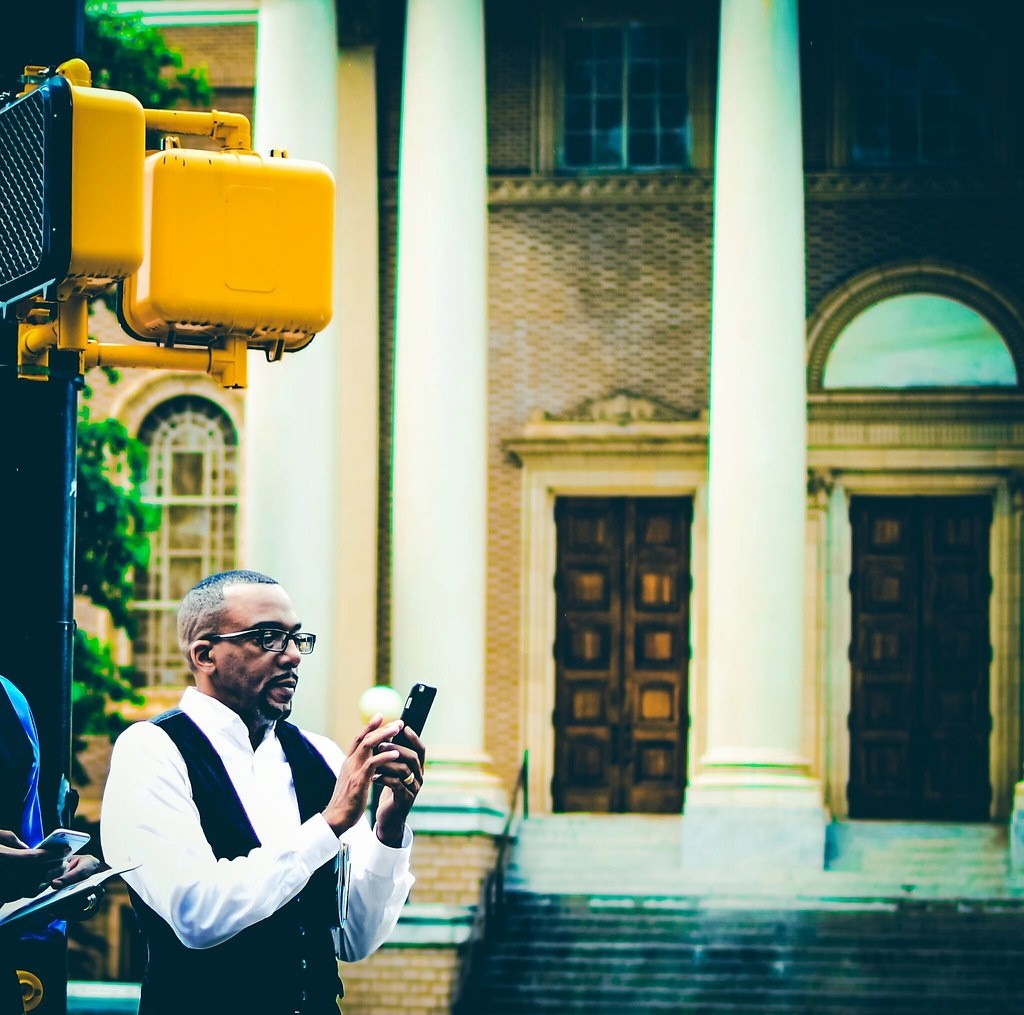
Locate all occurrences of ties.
[245,713,271,750]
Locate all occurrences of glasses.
[203,623,317,655]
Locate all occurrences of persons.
[1,675,106,1014]
[99,572,425,1015]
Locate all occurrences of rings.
[84,893,92,911]
[90,892,98,907]
[403,772,415,785]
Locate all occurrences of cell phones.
[380,683,437,777]
[32,829,92,859]
